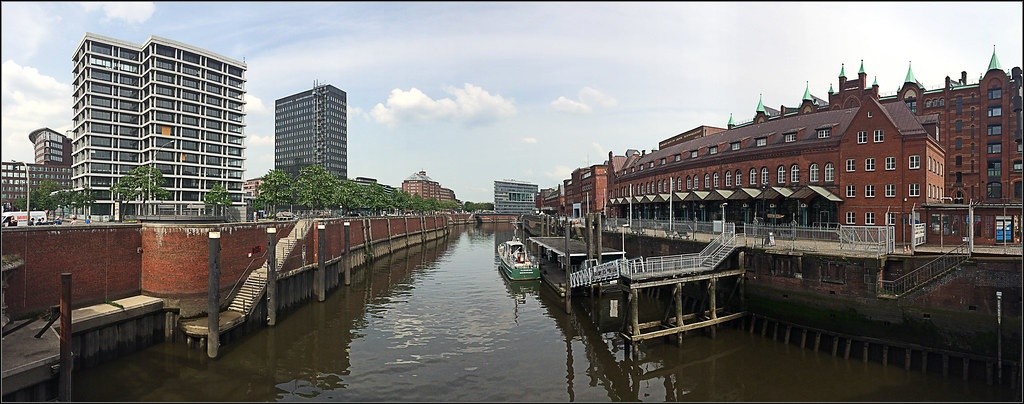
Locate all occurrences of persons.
[70,216,93,226]
[8,217,18,225]
[36,218,43,225]
[41,218,46,224]
[27,218,35,226]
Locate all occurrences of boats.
[497,239,541,282]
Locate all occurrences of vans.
[1,211,47,225]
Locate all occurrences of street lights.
[12,159,30,226]
[290,171,306,219]
[148,140,175,209]
